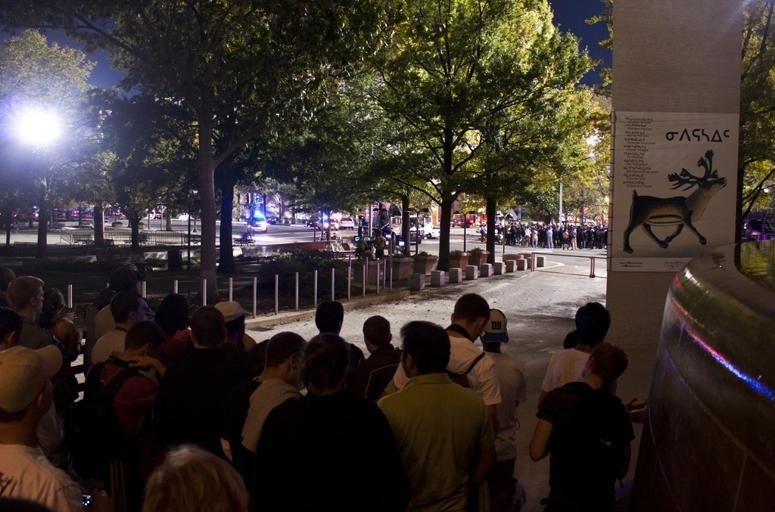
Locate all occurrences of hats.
[480,309,508,344]
[0,344,63,413]
[214,301,252,323]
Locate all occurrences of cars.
[388,213,438,247]
[318,217,341,236]
[340,217,356,232]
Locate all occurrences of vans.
[248,212,272,236]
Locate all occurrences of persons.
[534,300,646,418]
[528,341,637,511]
[2,262,527,512]
[477,217,608,252]
[354,233,374,261]
[373,230,388,261]
[562,330,578,349]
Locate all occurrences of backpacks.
[61,360,144,490]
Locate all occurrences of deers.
[623,150,727,254]
